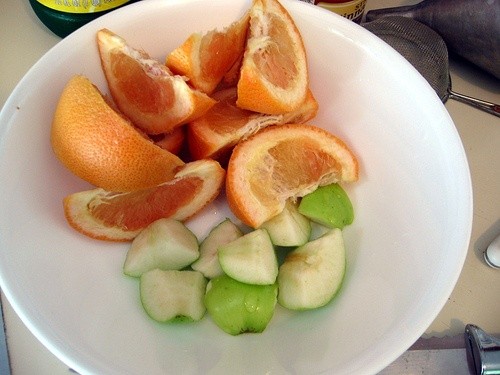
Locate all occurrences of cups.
[29,0,139,39]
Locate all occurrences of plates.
[0,0,474,373]
